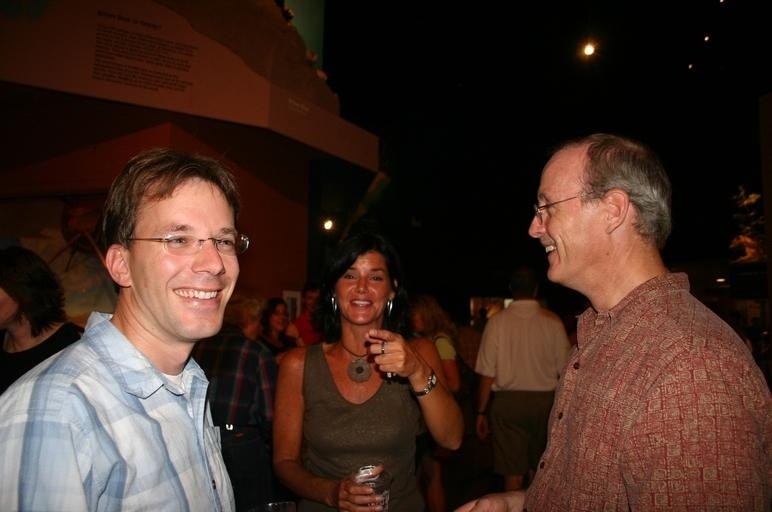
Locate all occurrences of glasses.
[124,233,250,257]
[534,195,579,225]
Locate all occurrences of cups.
[351,464,389,511]
[262,499,298,512]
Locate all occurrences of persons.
[0,244,84,397]
[455,133,772,512]
[0,149,249,512]
[194,234,572,512]
